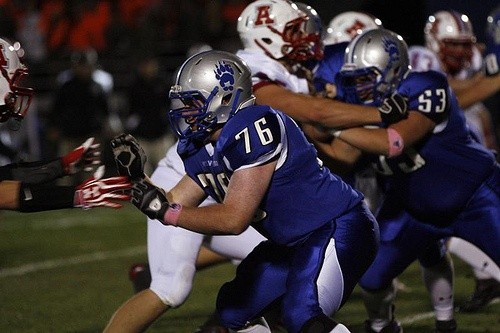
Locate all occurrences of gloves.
[110,133,152,192]
[74,166,133,211]
[130,180,182,227]
[378,91,409,128]
[62,137,102,177]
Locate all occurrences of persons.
[126,51,380,333]
[102,0,407,333]
[303,5,500,333]
[0,38,132,213]
[0,0,253,158]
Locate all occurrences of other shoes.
[128,263,151,290]
[435,319,457,333]
[348,319,403,333]
[462,278,500,313]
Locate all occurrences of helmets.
[424,10,473,54]
[236,0,308,60]
[485,8,500,47]
[339,29,410,94]
[0,38,21,106]
[295,2,322,40]
[169,50,256,124]
[323,12,379,47]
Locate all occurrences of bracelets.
[327,129,342,137]
[164,203,183,227]
